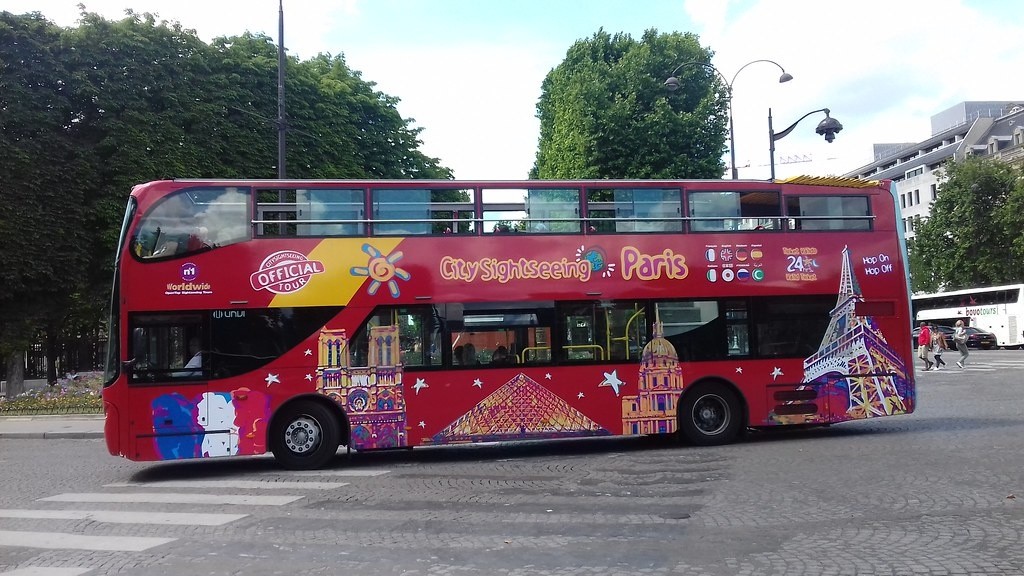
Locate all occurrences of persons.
[489,346,508,363]
[930,325,948,371]
[918,322,933,371]
[494,224,509,233]
[954,320,969,368]
[166,339,202,377]
[454,343,477,366]
[142,209,188,259]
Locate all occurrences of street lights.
[768,103,846,180]
[664,60,792,179]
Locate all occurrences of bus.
[99,178,917,470]
[911,284,1024,351]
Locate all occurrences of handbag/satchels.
[952,327,970,345]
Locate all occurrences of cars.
[911,325,955,350]
[941,327,997,351]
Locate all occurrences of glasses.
[933,328,938,330]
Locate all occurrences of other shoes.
[962,366,966,369]
[922,368,929,371]
[942,362,947,369]
[933,367,939,370]
[956,362,963,368]
[928,362,933,369]
